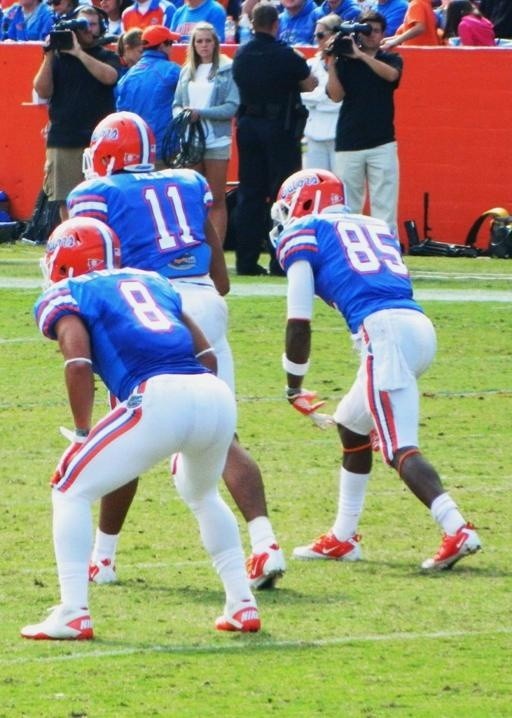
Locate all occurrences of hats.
[141,24,181,49]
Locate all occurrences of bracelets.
[286,387,301,394]
[76,429,89,437]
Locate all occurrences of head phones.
[63,6,111,34]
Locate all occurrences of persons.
[324,10,403,239]
[442,0,498,46]
[101,1,124,35]
[19,217,260,639]
[170,1,227,44]
[114,26,182,170]
[118,28,148,77]
[278,0,318,44]
[270,168,483,574]
[172,20,240,247]
[0,0,80,40]
[362,1,409,36]
[380,1,449,53]
[304,0,361,46]
[33,5,123,245]
[238,1,261,42]
[299,15,344,173]
[120,1,178,40]
[231,7,320,276]
[67,112,285,589]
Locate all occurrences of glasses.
[313,31,331,38]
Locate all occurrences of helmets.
[268,166,347,251]
[39,216,120,284]
[80,110,156,182]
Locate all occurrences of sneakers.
[270,260,284,275]
[293,530,361,562]
[246,543,286,590]
[87,558,118,587]
[19,605,95,641]
[238,263,267,276]
[214,598,260,633]
[421,521,482,574]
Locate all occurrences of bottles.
[239,13,250,44]
[223,15,236,44]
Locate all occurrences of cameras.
[326,22,371,54]
[43,18,89,52]
